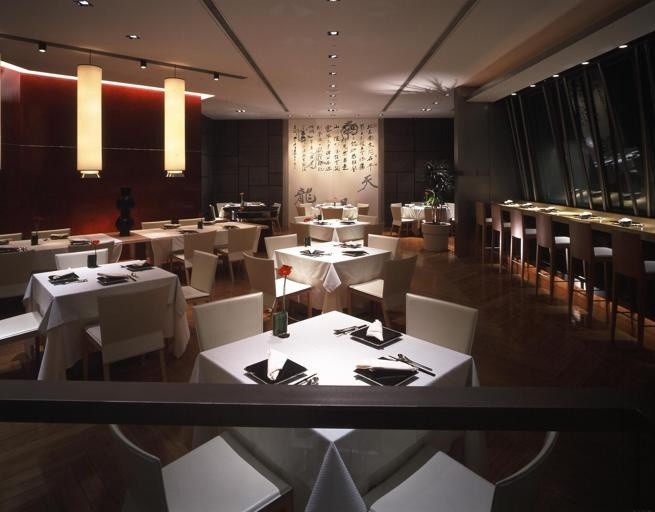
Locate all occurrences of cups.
[29,231,40,246]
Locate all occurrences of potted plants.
[422,160,455,252]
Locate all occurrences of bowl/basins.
[501,197,641,228]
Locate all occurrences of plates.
[312,216,358,226]
[47,262,156,286]
[0,233,90,252]
[299,240,368,258]
[243,324,435,387]
[163,222,242,234]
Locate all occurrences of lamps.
[0,32,247,184]
